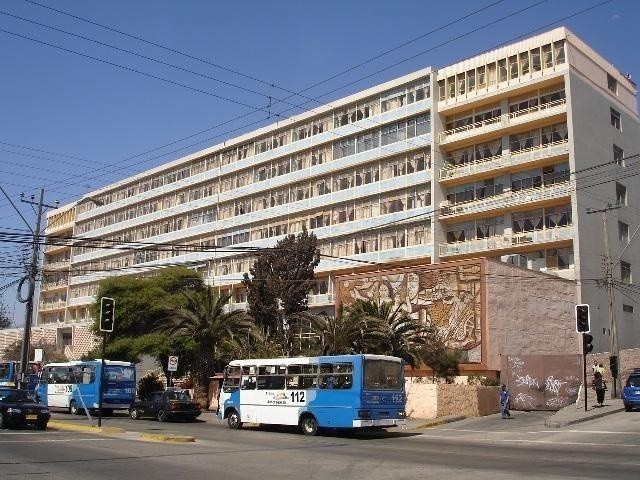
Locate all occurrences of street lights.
[17,196,103,389]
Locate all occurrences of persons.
[500,383,512,420]
[226,366,397,390]
[593,372,607,408]
[339,272,481,366]
[596,364,607,391]
[591,361,598,391]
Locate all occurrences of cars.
[129,391,202,422]
[0,385,50,429]
[622,372,640,410]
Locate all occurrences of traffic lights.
[102,299,113,329]
[577,306,588,332]
[610,357,617,371]
[585,334,593,352]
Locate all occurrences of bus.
[34,361,136,415]
[218,354,406,436]
